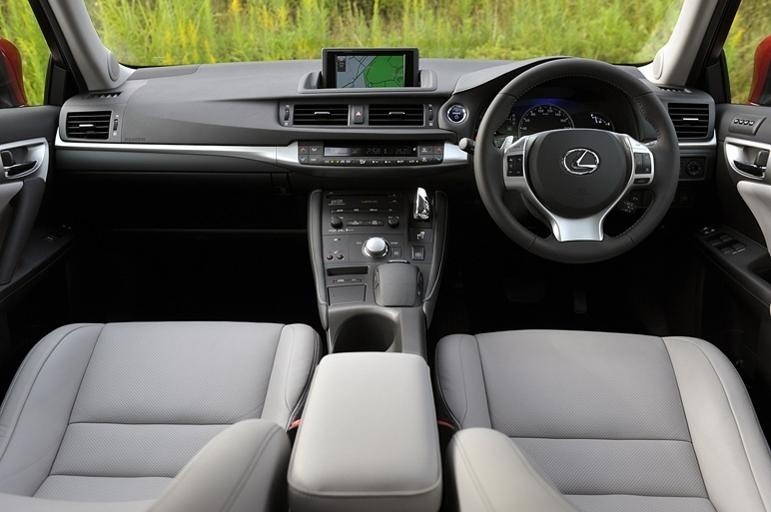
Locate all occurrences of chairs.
[434,322,771,510]
[0,318,326,512]
